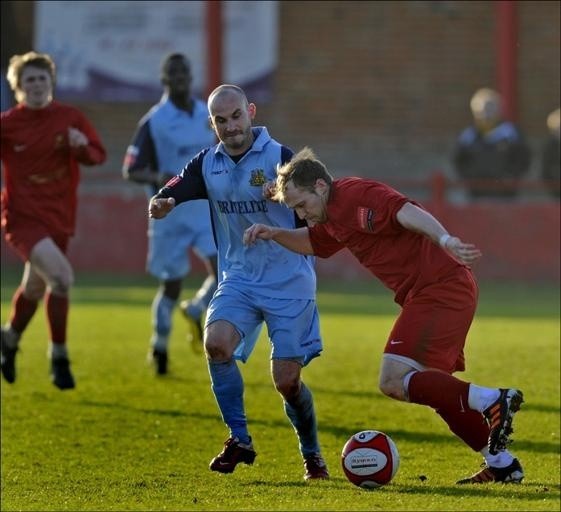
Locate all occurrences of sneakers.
[456,459,524,484]
[484,388,523,455]
[0,330,17,382]
[52,355,74,389]
[210,437,255,472]
[302,452,329,480]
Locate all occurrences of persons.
[542,109,560,199]
[148,83,329,479]
[454,85,527,202]
[0,51,107,390]
[122,51,219,375]
[243,147,524,485]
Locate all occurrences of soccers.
[342,430,400,489]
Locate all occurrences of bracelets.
[437,235,449,251]
[156,171,163,183]
